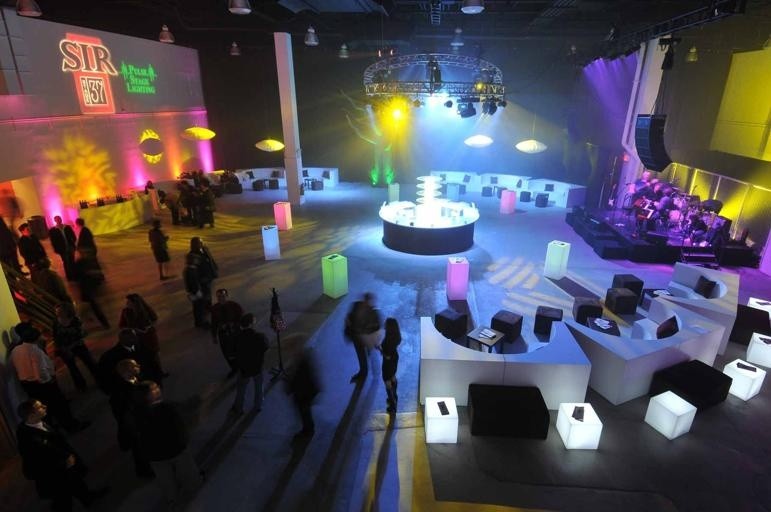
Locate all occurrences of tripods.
[269,288,286,382]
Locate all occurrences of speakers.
[635,114,671,172]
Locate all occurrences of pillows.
[302,169,329,179]
[463,174,471,182]
[490,176,497,184]
[544,184,554,192]
[695,275,717,299]
[516,179,522,188]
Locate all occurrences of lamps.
[228,0,253,15]
[457,94,500,118]
[338,41,351,59]
[304,23,320,46]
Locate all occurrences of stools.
[434,273,644,343]
[555,401,604,450]
[424,396,460,445]
[481,186,549,208]
[643,297,771,441]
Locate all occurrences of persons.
[184,236,218,331]
[348,293,380,381]
[229,313,269,410]
[3,215,111,330]
[2,294,161,509]
[374,318,401,413]
[281,347,319,435]
[149,220,170,280]
[144,169,239,228]
[626,170,707,244]
[210,289,245,376]
